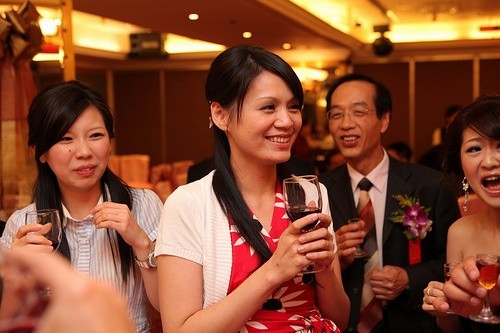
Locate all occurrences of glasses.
[322,106,378,120]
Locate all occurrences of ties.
[356,178,384,333]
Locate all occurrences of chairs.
[107,154,195,205]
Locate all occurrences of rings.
[427,287,432,295]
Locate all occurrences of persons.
[0,80,164,333]
[184,101,462,184]
[2,243,138,333]
[152,44,350,333]
[422,94,500,332]
[315,72,463,333]
[431,254,500,319]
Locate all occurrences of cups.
[25,209,61,253]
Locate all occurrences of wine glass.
[283,175,325,275]
[348,218,370,259]
[469,253,500,325]
[444,262,461,315]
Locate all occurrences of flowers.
[388,194,433,243]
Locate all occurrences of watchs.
[133,249,158,270]
